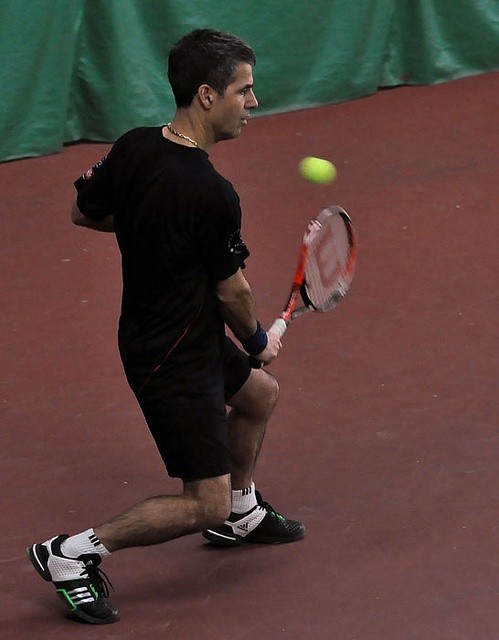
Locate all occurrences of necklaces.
[162,123,207,155]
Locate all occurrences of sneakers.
[26,533,121,624]
[202,490,306,547]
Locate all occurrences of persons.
[26,28,307,625]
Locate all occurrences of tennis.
[298,155,337,185]
